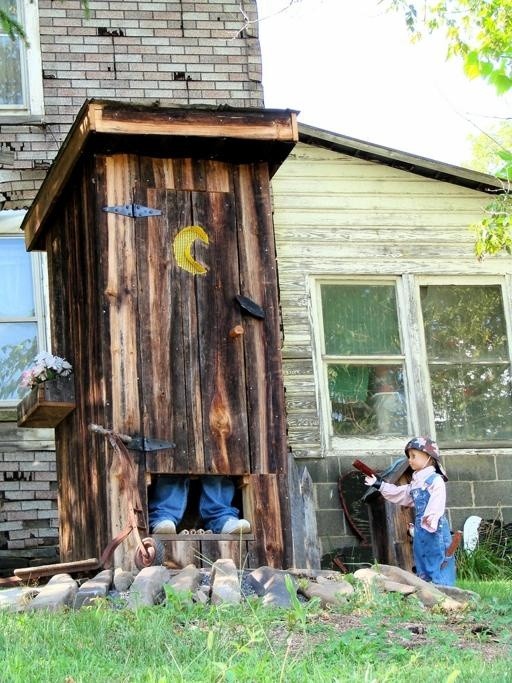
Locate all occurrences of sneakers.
[151,520,177,534]
[221,518,251,534]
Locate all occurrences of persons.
[365,438,457,587]
[148,476,251,533]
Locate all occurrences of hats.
[404,435,449,482]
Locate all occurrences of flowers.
[16,348,76,390]
